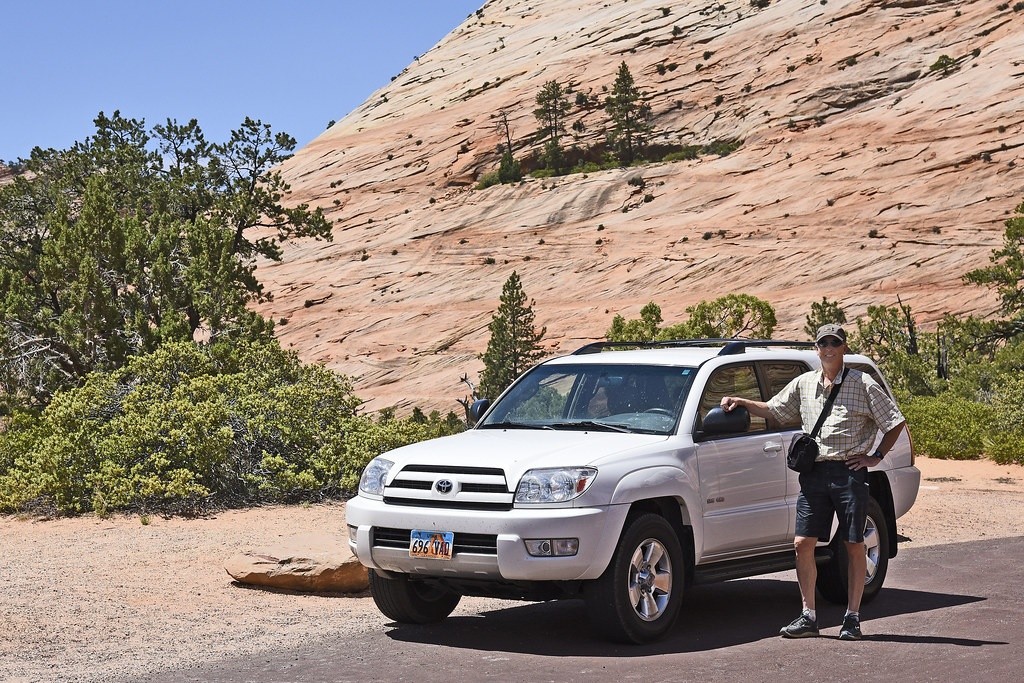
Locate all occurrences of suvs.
[344,340,920,644]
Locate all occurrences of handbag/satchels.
[787,434,818,473]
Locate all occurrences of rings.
[857,461,860,465]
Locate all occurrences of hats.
[815,324,846,343]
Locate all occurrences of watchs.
[873,451,884,460]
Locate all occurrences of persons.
[720,323,906,640]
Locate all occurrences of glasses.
[817,339,842,348]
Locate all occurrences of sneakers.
[839,613,862,640]
[779,613,819,638]
